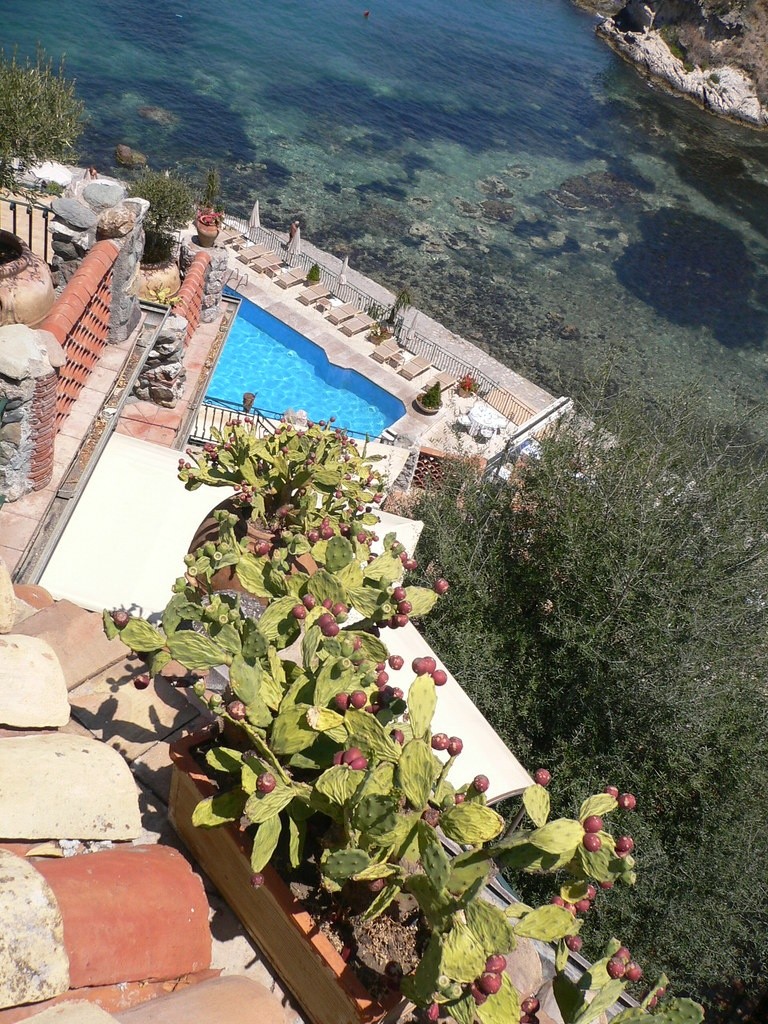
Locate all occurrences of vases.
[196,215,219,248]
[370,335,377,343]
[459,388,470,397]
[1,230,56,330]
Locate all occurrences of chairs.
[214,227,243,249]
[298,283,331,306]
[328,301,377,336]
[373,340,404,362]
[402,353,433,381]
[448,389,515,447]
[238,243,284,275]
[278,266,306,290]
[425,369,456,393]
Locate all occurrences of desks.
[469,405,500,437]
[387,353,405,370]
[232,238,248,253]
[316,298,332,315]
[266,264,282,280]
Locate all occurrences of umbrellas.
[336,256,348,296]
[288,228,300,266]
[248,200,260,227]
[404,311,419,347]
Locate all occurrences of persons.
[84,166,97,180]
[287,221,299,247]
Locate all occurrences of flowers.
[196,195,224,225]
[370,327,381,336]
[460,372,480,392]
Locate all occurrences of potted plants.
[365,288,414,343]
[126,165,203,303]
[102,392,704,1024]
[416,381,443,413]
[306,263,321,287]
